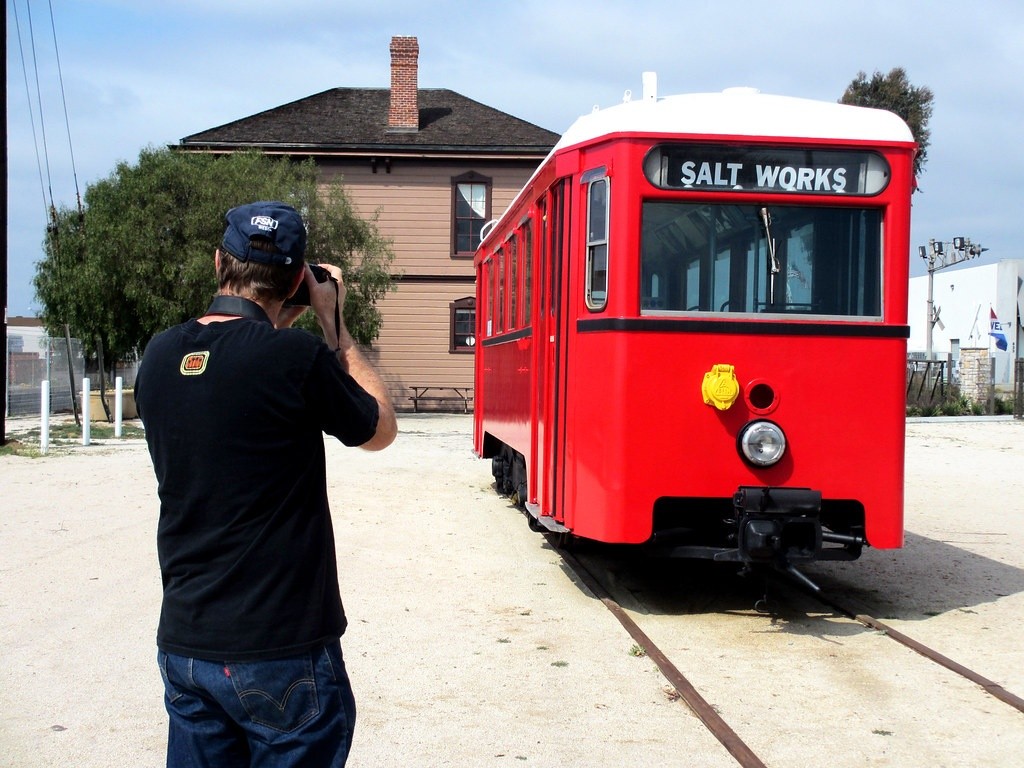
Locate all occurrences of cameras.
[282,264,331,308]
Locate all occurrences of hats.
[221,200,306,266]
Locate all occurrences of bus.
[473,70,919,577]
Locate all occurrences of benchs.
[409,386,473,414]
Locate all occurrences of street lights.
[918,236,990,359]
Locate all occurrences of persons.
[133,200,397,768]
